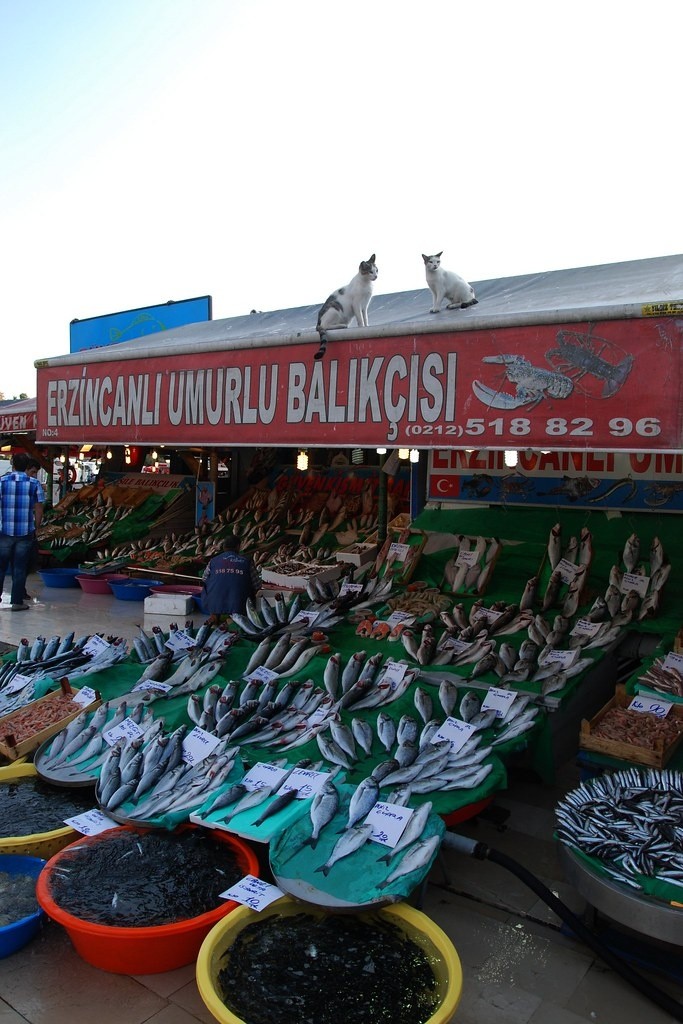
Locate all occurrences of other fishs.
[520,522,672,628]
[316,701,683,888]
[43,699,300,826]
[400,599,683,703]
[301,775,442,892]
[84,546,129,565]
[187,650,420,753]
[229,584,346,682]
[130,483,316,561]
[0,633,129,691]
[41,493,134,548]
[309,479,501,595]
[133,618,239,697]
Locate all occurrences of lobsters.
[472,353,573,410]
[545,322,634,402]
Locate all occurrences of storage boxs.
[578,684,683,770]
[387,512,411,532]
[142,593,194,616]
[364,530,390,546]
[255,590,293,609]
[0,677,102,758]
[263,561,342,589]
[337,542,378,568]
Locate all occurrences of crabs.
[496,472,535,503]
[461,473,492,498]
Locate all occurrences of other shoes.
[23,594,31,600]
[12,603,29,611]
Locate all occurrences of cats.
[314,253,378,360]
[422,250,478,313]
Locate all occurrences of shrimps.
[385,588,451,619]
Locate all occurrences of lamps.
[78,444,94,453]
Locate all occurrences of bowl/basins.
[196,894,463,1024]
[192,593,210,614]
[75,572,128,594]
[107,579,165,600]
[0,854,48,959]
[0,763,83,860]
[36,825,260,976]
[149,584,203,595]
[36,568,86,588]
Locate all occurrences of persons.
[201,537,261,615]
[0,453,44,611]
